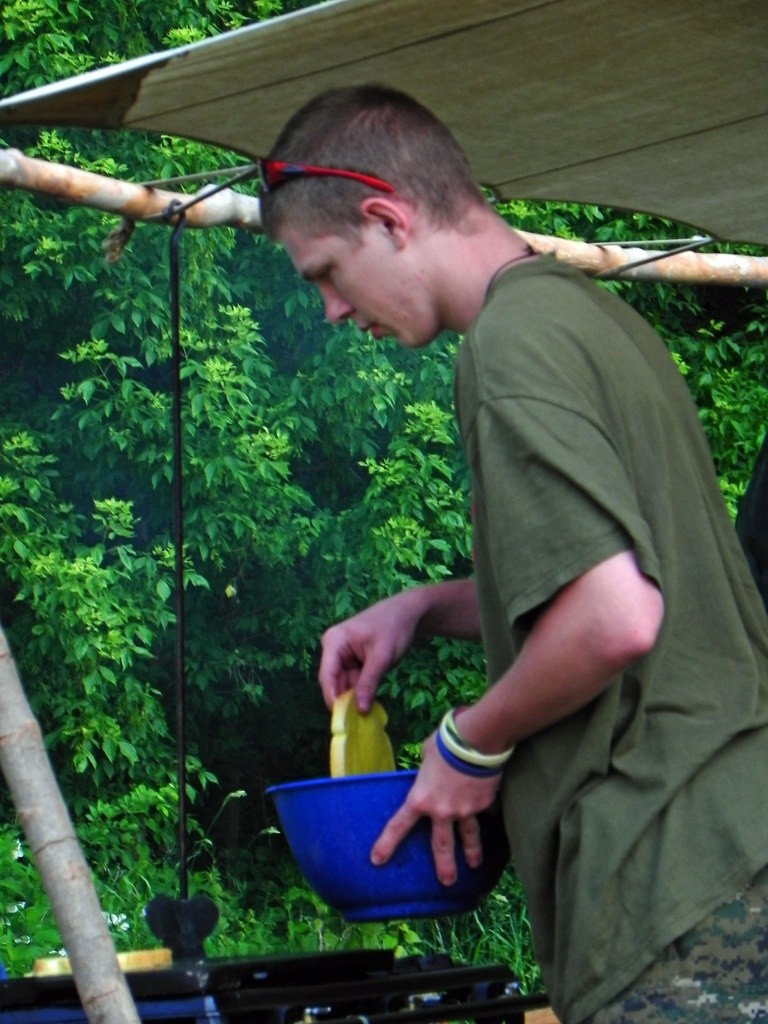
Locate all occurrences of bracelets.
[436,703,518,778]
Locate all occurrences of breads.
[328,689,396,779]
[32,947,172,978]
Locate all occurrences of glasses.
[256,158,395,194]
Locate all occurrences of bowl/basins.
[261,770,515,922]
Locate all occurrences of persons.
[258,78,767,1024]
